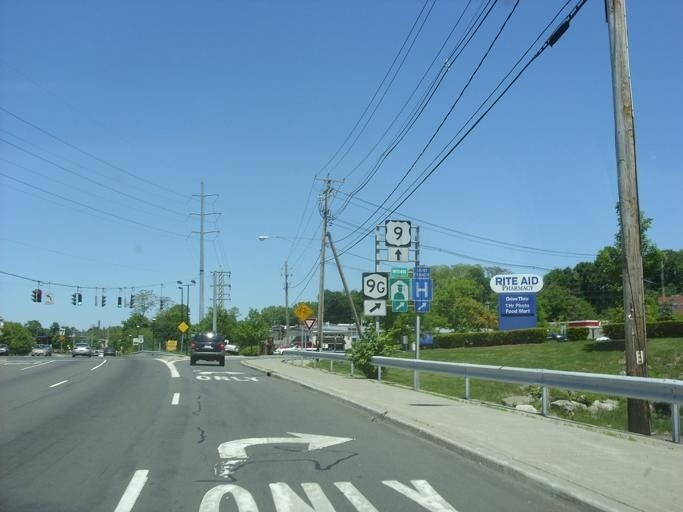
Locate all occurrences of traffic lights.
[29,288,166,312]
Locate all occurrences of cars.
[30,343,52,356]
[72,342,92,357]
[0,343,10,356]
[190,329,225,366]
[103,347,117,357]
